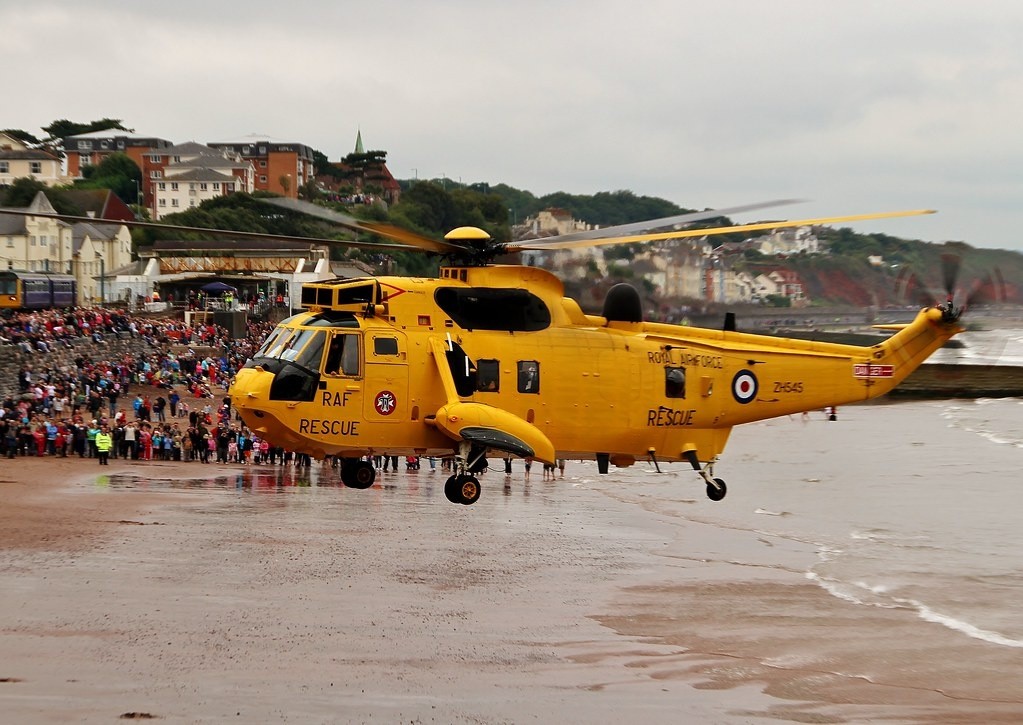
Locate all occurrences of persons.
[440,458,455,470]
[123,422,137,460]
[429,459,437,471]
[405,456,420,470]
[373,456,398,473]
[503,458,513,475]
[525,459,532,478]
[543,459,565,480]
[326,192,381,206]
[0,286,311,469]
[96,428,112,465]
[322,457,338,470]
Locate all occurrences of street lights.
[411,168,417,179]
[99,257,104,308]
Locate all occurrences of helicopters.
[0,200,1005,505]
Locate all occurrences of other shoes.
[375,463,459,474]
[216,461,219,463]
[201,461,204,464]
[206,461,209,464]
[225,461,228,464]
[240,461,311,467]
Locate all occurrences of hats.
[174,422,178,425]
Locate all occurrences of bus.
[0,268,78,312]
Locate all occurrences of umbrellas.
[201,282,237,291]
